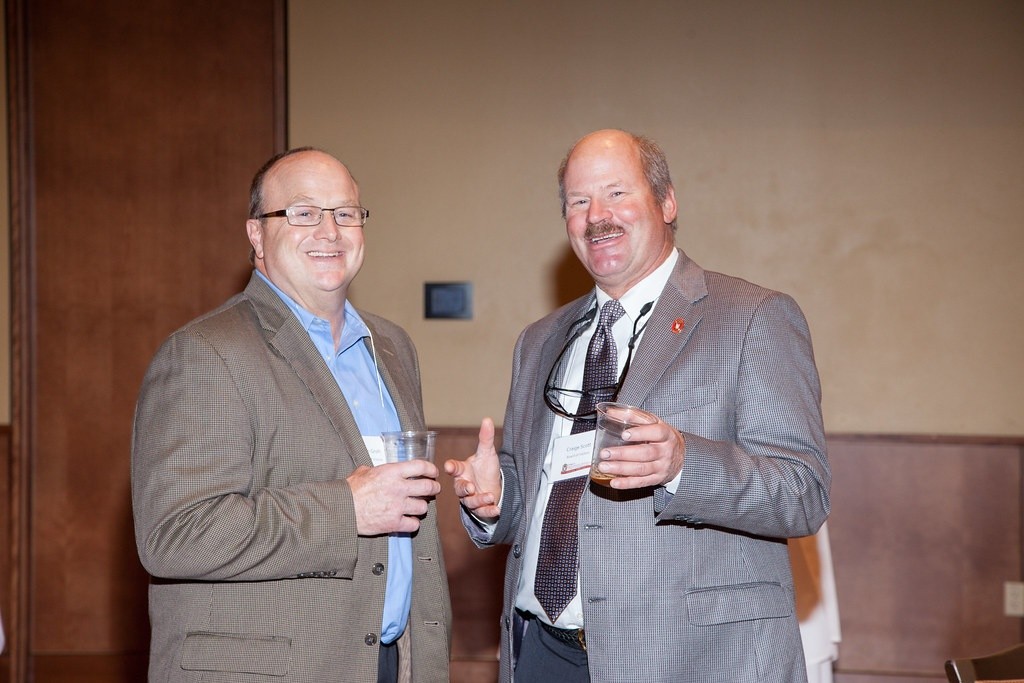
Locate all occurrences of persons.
[131,147,452,682]
[443,127,832,683]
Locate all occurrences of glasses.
[252,206,370,227]
[543,301,654,424]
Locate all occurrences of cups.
[589,401,659,487]
[382,431,437,503]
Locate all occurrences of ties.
[533,298,626,625]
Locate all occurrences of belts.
[539,620,587,650]
